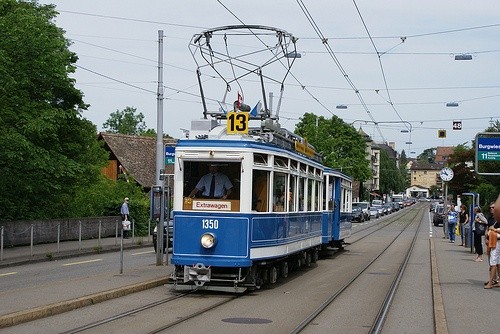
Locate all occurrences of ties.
[209,175,216,199]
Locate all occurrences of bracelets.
[479,221,482,223]
[494,229,497,232]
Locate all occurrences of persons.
[472,207,488,262]
[457,205,468,247]
[484,195,500,289]
[442,203,458,243]
[254,174,267,212]
[120,197,131,239]
[273,187,304,212]
[188,163,233,200]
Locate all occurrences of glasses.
[126,200,129,201]
[489,207,494,209]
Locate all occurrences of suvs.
[153,210,173,253]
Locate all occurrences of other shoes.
[449,240,451,242]
[124,236,129,239]
[484,276,500,289]
[473,257,483,262]
[452,240,454,243]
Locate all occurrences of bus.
[164,91,352,293]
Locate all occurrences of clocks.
[440,167,454,182]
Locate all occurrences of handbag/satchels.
[122,219,132,231]
[475,222,486,236]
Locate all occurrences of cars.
[418,197,431,202]
[352,194,416,223]
[430,195,451,226]
